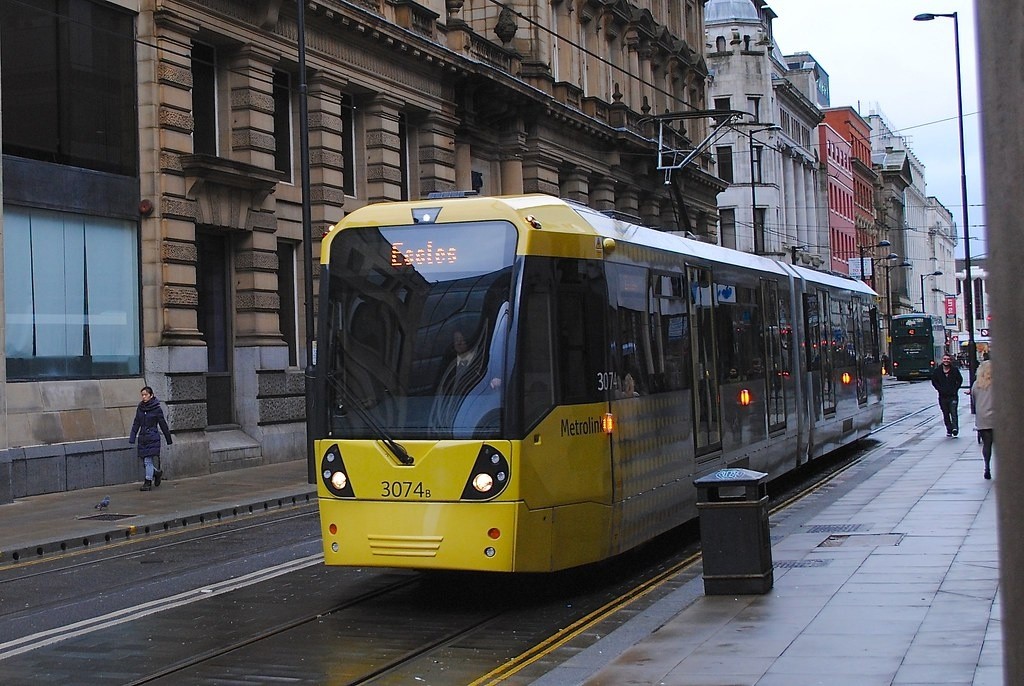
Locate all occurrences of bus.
[306,111,883,575]
[888,313,945,379]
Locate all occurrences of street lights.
[915,11,978,414]
[748,126,783,255]
[857,239,910,359]
[919,271,944,314]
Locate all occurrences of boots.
[153,469,163,486]
[140,479,152,491]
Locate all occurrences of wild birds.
[95,496,110,511]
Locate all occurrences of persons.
[881,351,889,375]
[812,353,832,402]
[970,360,994,480]
[435,327,503,396]
[931,354,963,438]
[726,358,765,383]
[128,386,173,491]
[957,353,990,395]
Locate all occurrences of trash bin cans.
[693,468,774,595]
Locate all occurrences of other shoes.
[984,469,991,479]
[947,432,951,436]
[952,429,959,437]
